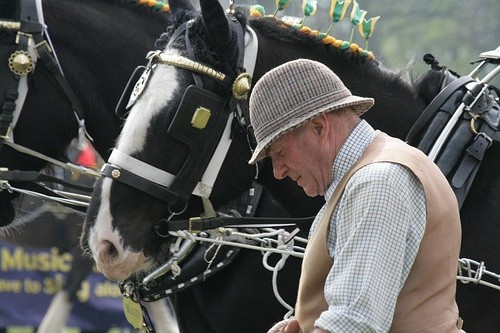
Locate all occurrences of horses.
[80,0,500,333]
[0,0,306,333]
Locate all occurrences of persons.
[248,58,466,333]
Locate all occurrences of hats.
[248,59,375,165]
